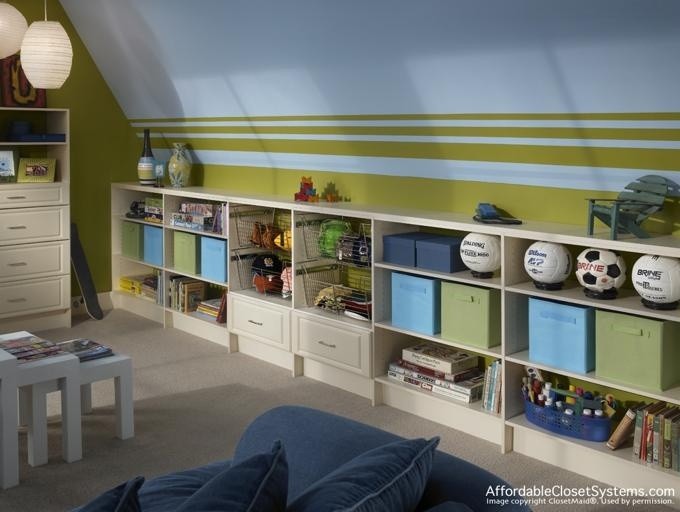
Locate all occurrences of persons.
[294,176,339,203]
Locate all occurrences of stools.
[0,329,135,490]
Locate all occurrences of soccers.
[574,249,626,291]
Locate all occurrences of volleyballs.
[632,255,680,303]
[460,233,501,273]
[524,241,571,283]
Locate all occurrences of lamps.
[0,0,74,90]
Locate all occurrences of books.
[608,402,680,471]
[168,201,227,235]
[1,330,113,361]
[385,339,502,414]
[165,274,226,323]
[118,268,162,305]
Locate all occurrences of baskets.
[234,208,371,323]
[523,399,611,443]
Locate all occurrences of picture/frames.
[16,158,56,182]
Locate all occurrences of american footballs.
[274,230,292,251]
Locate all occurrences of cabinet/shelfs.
[110,178,680,512]
[0,107,71,332]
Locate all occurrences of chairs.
[64,406,531,512]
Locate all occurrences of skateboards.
[70,222,103,319]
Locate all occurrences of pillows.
[64,432,439,512]
